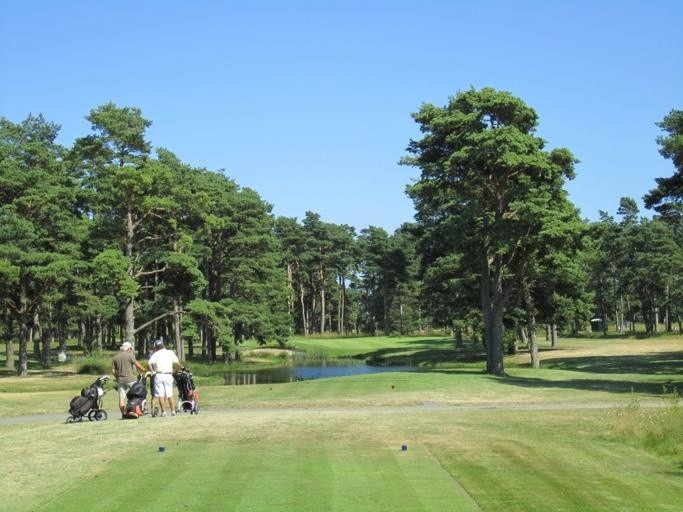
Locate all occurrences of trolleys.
[65,376,110,421]
[123,372,153,419]
[173,367,200,415]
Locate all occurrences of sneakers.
[159,412,166,417]
[170,411,175,416]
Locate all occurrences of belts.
[156,371,171,374]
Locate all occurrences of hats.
[119,342,131,351]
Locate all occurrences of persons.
[148,340,181,417]
[148,347,157,413]
[112,342,146,419]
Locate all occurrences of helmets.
[153,340,162,348]
